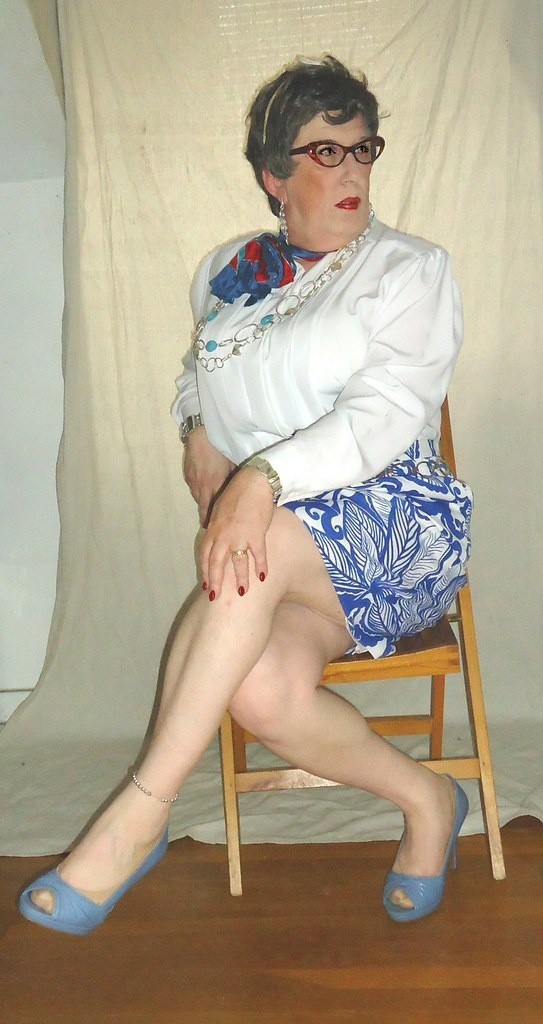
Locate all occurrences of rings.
[232,549,248,557]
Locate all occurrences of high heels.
[18,815,170,938]
[379,771,470,924]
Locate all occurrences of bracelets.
[242,458,282,504]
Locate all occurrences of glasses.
[289,135,386,169]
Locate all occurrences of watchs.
[179,413,204,442]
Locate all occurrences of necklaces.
[193,202,374,372]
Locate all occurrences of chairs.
[218,397,507,896]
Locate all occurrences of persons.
[20,52,478,934]
